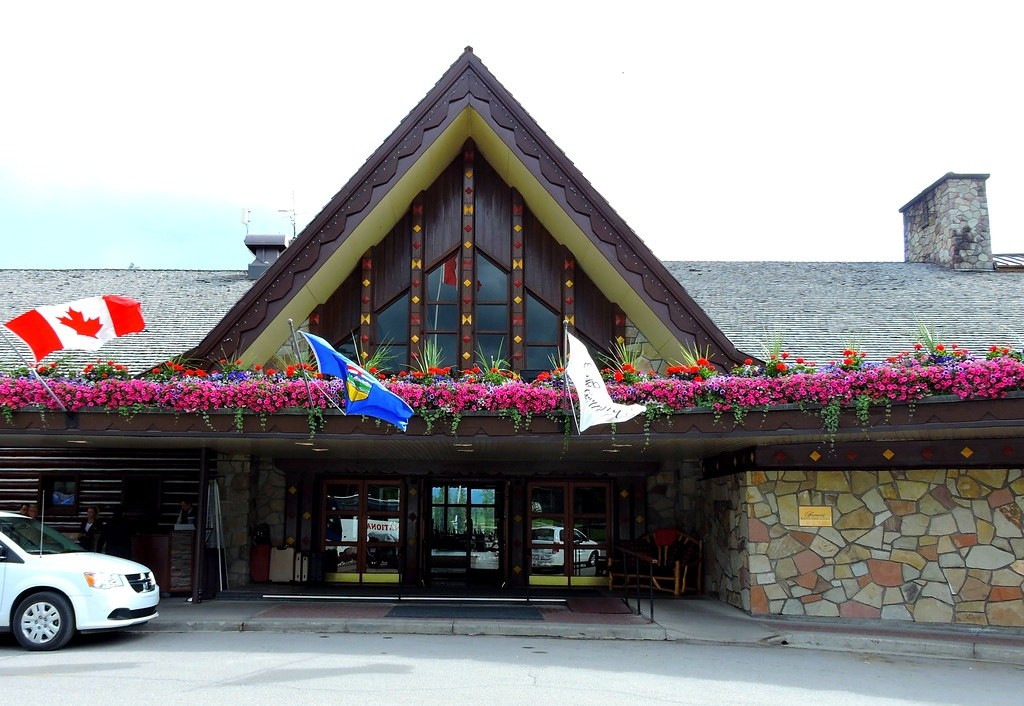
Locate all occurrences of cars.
[0,511,161,651]
[531,525,600,573]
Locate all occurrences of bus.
[328,495,401,557]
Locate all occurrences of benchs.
[526,571,618,607]
[325,572,404,603]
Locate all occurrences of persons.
[10,503,46,547]
[176,499,196,524]
[77,505,105,553]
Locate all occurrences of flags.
[301,331,415,433]
[565,332,647,433]
[4,295,145,368]
